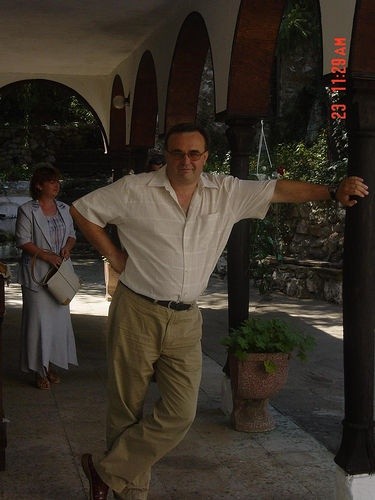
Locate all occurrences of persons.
[70,124,369,500]
[14,165,81,390]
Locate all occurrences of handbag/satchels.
[31,249,81,305]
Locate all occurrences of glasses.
[167,151,206,161]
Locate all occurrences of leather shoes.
[81,454,109,500]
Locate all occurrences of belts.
[119,280,193,311]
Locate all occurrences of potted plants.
[222,315,316,433]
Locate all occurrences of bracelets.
[329,186,336,199]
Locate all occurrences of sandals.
[35,372,50,389]
[47,367,60,383]
